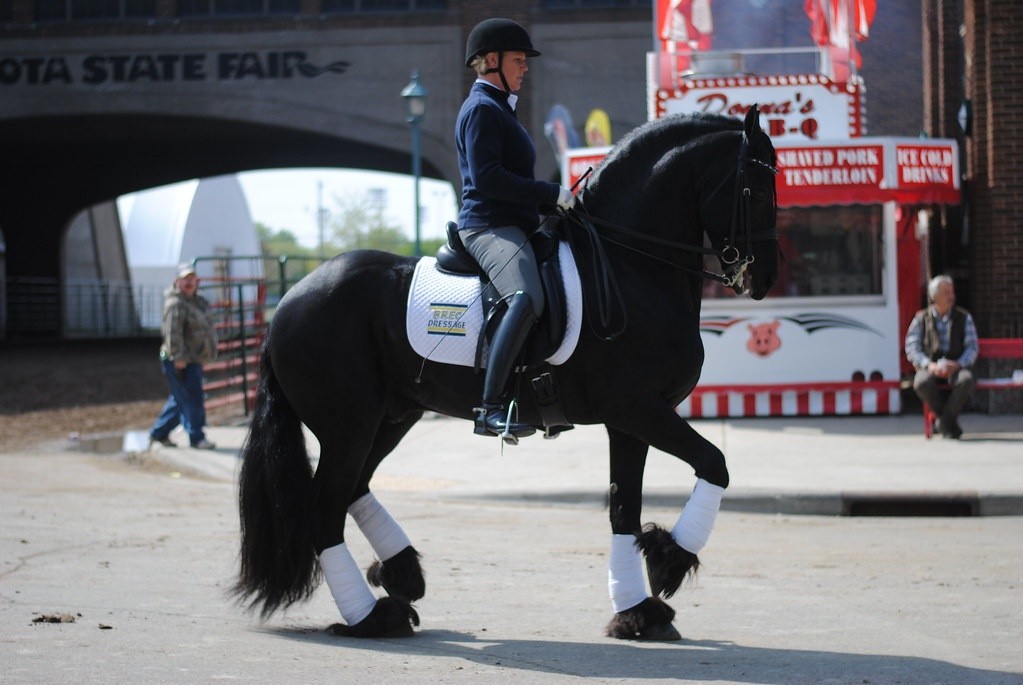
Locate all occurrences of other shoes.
[939,415,963,439]
[193,440,214,449]
[149,435,176,446]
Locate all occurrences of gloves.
[557,186,576,212]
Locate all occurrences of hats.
[177,263,195,278]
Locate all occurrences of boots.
[473,291,537,437]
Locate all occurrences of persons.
[905,274,979,436]
[149,262,218,450]
[453,17,575,438]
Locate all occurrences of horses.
[221,102,783,644]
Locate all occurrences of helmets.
[465,18,542,68]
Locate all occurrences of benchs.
[901,338,1023,440]
